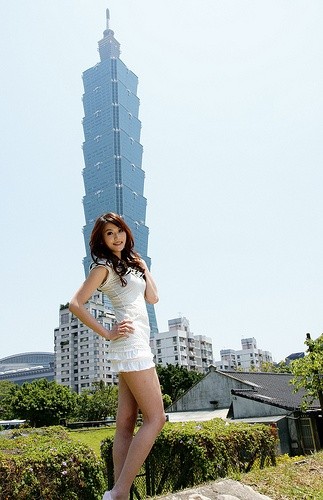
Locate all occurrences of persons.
[67,210,171,500]
